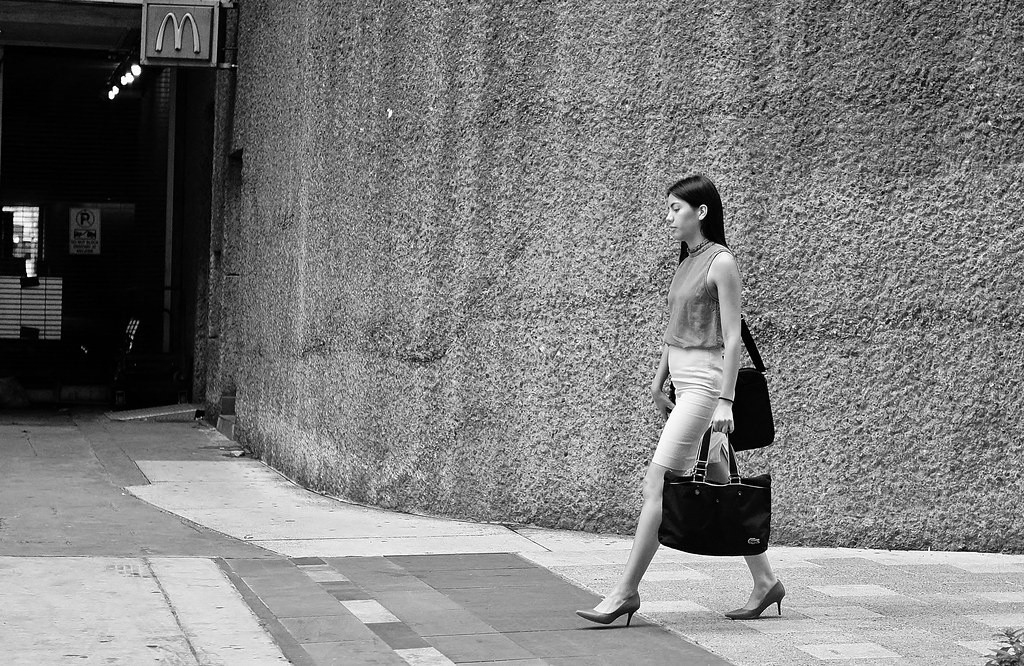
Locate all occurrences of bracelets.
[717,396,735,404]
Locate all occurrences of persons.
[575,176,786,625]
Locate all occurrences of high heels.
[726,579,785,620]
[575,591,640,628]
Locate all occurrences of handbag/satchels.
[657,422,771,557]
[669,368,774,452]
